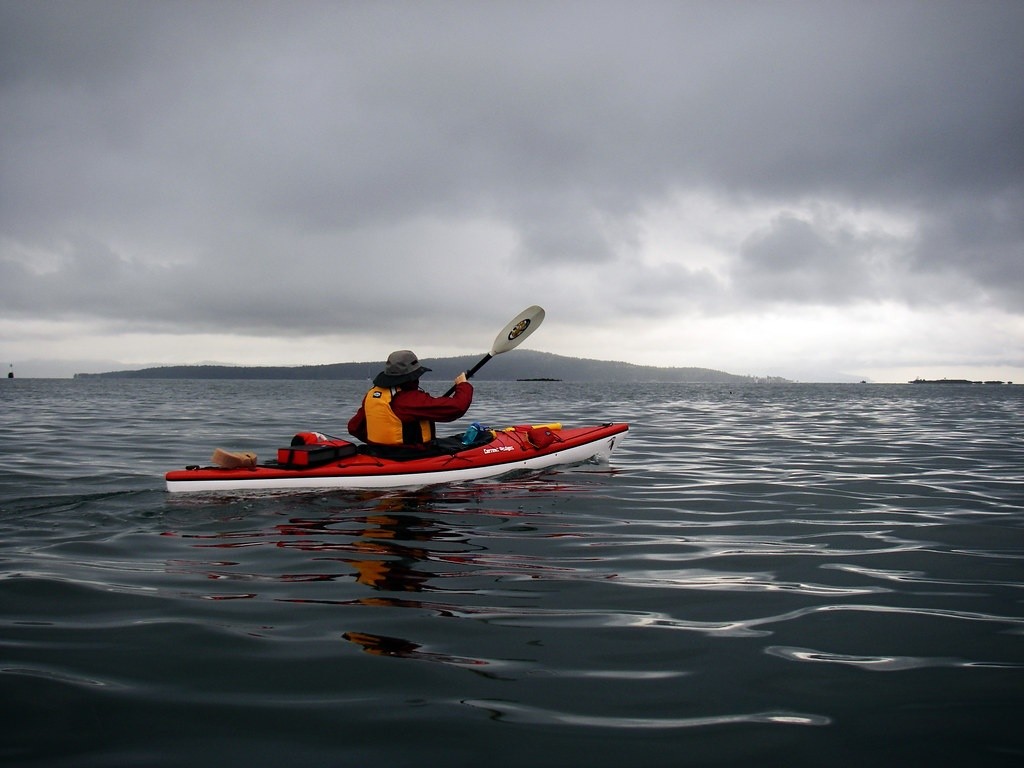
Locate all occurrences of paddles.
[442,305,545,397]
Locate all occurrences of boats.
[166,424,628,491]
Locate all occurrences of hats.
[373,350,433,388]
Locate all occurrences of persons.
[348,350,473,445]
